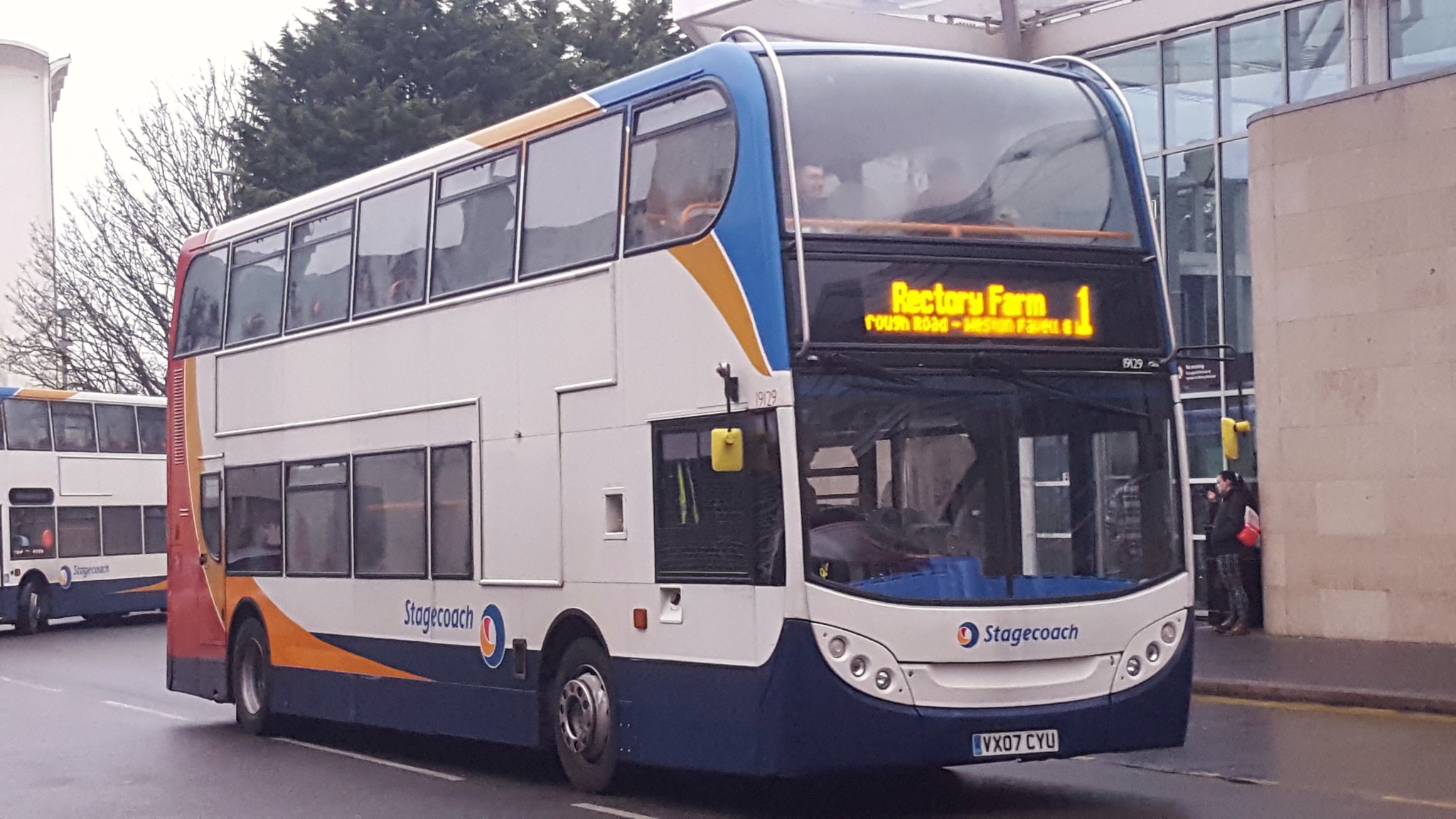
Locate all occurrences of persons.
[795,164,829,220]
[1105,464,1253,637]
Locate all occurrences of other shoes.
[1224,621,1252,637]
[1212,619,1235,632]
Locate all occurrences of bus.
[0,385,169,638]
[162,26,1252,789]
